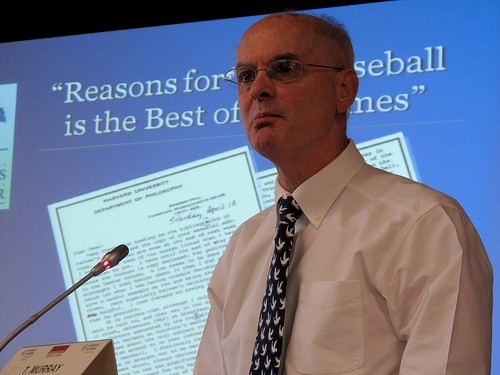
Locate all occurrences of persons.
[193,13,494,375]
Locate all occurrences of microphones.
[0,244,130,352]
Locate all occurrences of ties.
[248,196,303,375]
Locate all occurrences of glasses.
[223,58,344,91]
